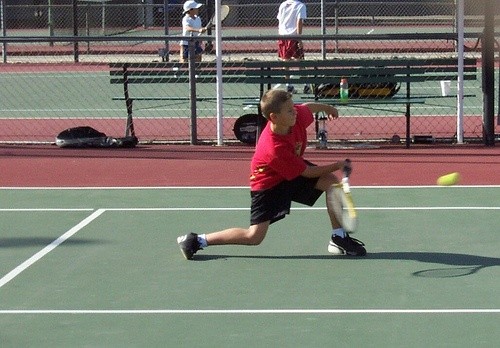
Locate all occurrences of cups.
[440,80,452,97]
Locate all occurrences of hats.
[182,0,203,14]
[287,86,298,94]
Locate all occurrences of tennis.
[436,172,459,186]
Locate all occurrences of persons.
[277,0,313,93]
[181,1,204,62]
[177,90,366,257]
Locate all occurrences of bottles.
[340,78,351,102]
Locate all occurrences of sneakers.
[328,232,367,257]
[304,85,312,94]
[177,230,202,259]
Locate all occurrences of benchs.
[109,57,477,149]
